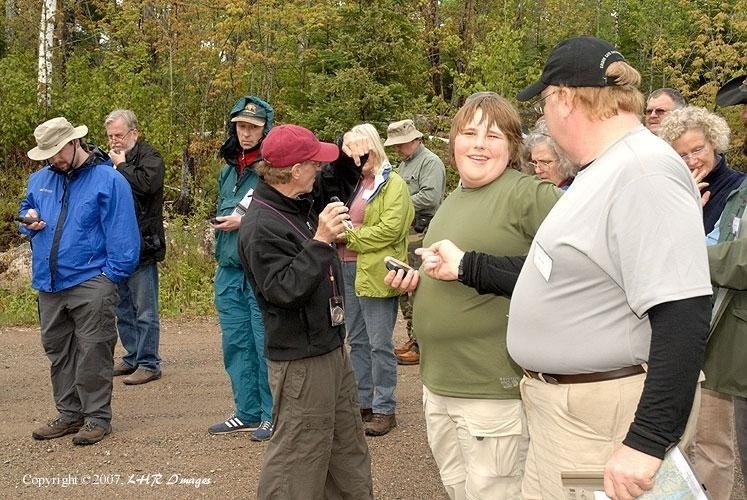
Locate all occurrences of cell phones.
[15,216,44,224]
[331,194,352,221]
[211,217,224,225]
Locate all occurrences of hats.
[384,120,424,147]
[516,36,630,101]
[231,103,266,128]
[27,117,88,161]
[715,75,747,106]
[262,125,340,168]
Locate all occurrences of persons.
[99,109,167,385]
[236,35,747,500]
[207,95,276,442]
[16,116,141,446]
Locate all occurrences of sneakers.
[208,415,260,434]
[73,418,112,444]
[249,421,272,440]
[360,408,396,435]
[33,415,83,439]
[113,361,136,376]
[395,343,420,365]
[123,369,161,384]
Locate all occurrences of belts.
[523,365,645,384]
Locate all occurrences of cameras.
[414,214,428,233]
[386,259,412,278]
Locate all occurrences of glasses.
[526,162,554,173]
[106,129,131,142]
[680,141,708,162]
[533,89,560,115]
